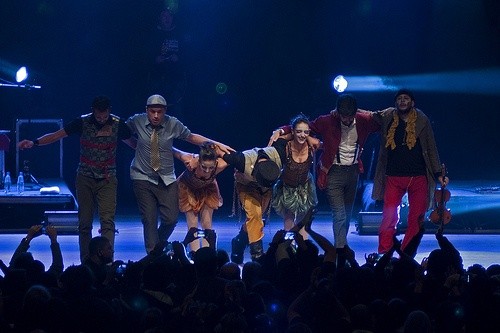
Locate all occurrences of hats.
[337,94,357,117]
[395,89,414,102]
[254,160,279,187]
[147,94,167,106]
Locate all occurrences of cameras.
[197,230,209,238]
[41,227,48,234]
[285,232,296,240]
[375,253,384,262]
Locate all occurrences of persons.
[169,141,230,252]
[0,224,500,333]
[272,112,324,254]
[18,97,137,264]
[125,95,237,255]
[267,95,376,267]
[184,133,320,264]
[330,89,449,260]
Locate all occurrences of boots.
[249,240,264,262]
[231,225,248,264]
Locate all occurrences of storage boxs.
[13,117,66,187]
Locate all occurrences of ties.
[150,128,160,172]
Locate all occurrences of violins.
[429,163,453,225]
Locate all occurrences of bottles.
[17,172,24,196]
[4,172,11,196]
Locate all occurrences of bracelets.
[33,140,39,146]
[279,130,282,136]
[26,238,29,242]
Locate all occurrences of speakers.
[358,212,385,235]
[44,211,80,236]
